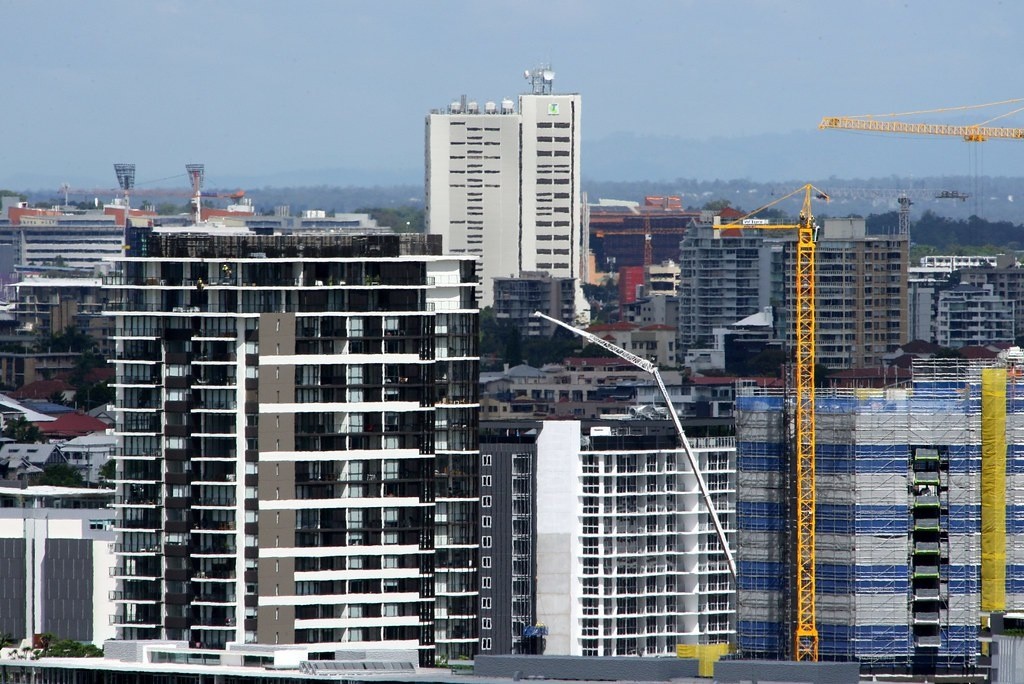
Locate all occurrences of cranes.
[834,185,965,237]
[818,97,1024,145]
[715,182,831,661]
